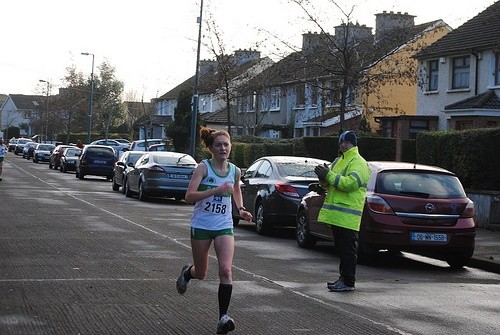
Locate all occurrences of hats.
[338,131,357,145]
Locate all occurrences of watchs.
[240,207,245,211]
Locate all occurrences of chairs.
[383,179,398,192]
[401,178,420,192]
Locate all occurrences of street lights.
[81,53,94,144]
[39,79,50,144]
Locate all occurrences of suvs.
[14,138,34,155]
[48,145,76,171]
[74,144,116,182]
[294,160,477,270]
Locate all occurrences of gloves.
[314,163,330,181]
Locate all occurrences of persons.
[0,137,8,182]
[77,139,83,148]
[176,127,254,335]
[314,130,371,291]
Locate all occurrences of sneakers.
[216,314,235,335]
[177,265,190,293]
[327,276,355,291]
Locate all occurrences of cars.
[232,156,335,234]
[22,142,39,160]
[60,148,82,173]
[111,151,147,194]
[32,144,57,163]
[124,151,200,201]
[7,137,20,152]
[31,135,47,142]
